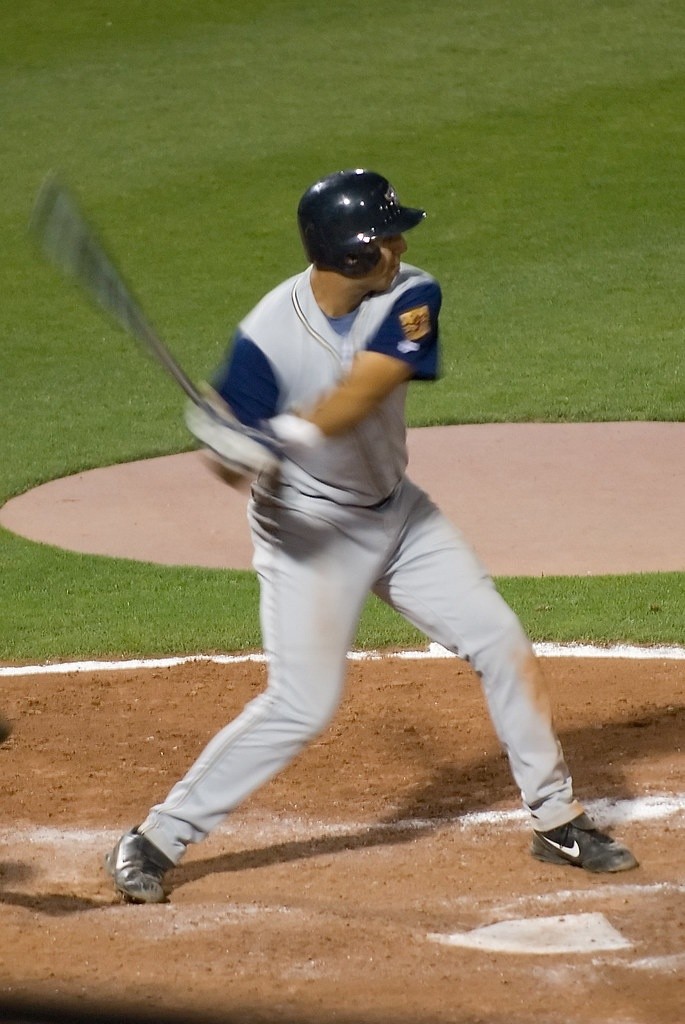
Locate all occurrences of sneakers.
[105,825,176,903]
[532,812,637,874]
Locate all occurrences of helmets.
[297,168,428,276]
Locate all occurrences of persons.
[104,169,637,903]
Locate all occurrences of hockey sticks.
[25,168,205,412]
